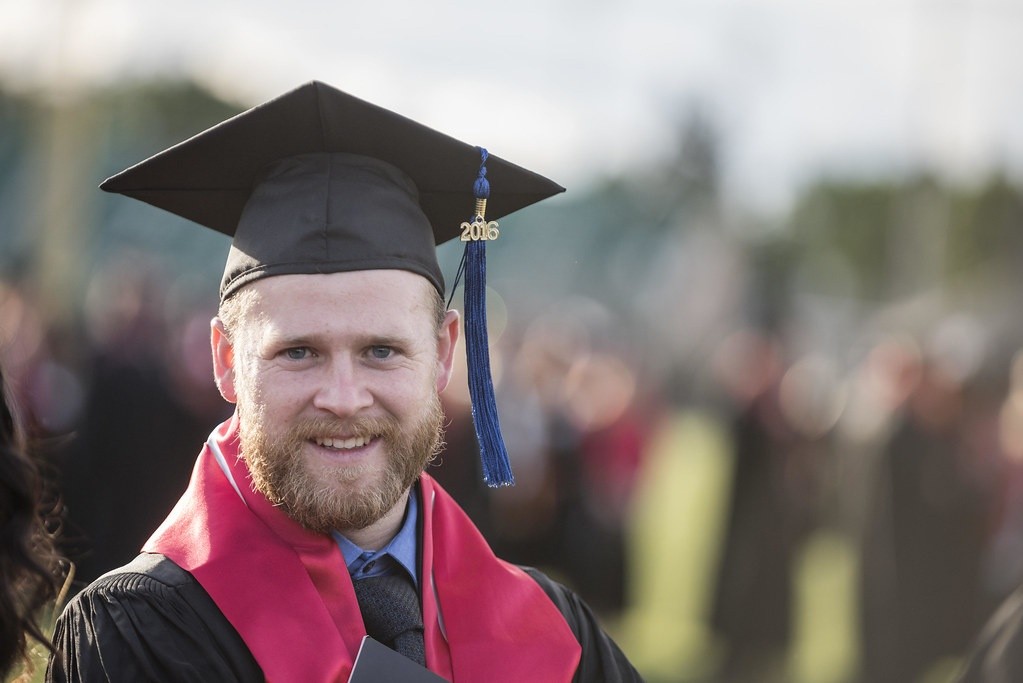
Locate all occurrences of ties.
[355,574,426,667]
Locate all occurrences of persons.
[0,252,1023,683]
[46,80,645,683]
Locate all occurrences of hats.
[98,80,567,487]
